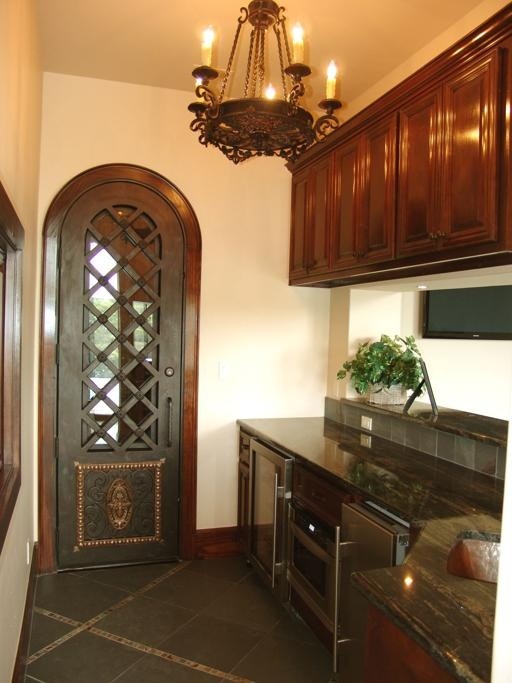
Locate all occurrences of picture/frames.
[403,359,442,416]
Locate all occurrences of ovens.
[282,459,358,661]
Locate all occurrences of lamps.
[186,1,342,163]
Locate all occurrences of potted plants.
[335,335,423,406]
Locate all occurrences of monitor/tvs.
[422,285,512,339]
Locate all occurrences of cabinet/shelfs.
[289,2,512,288]
[238,428,473,681]
